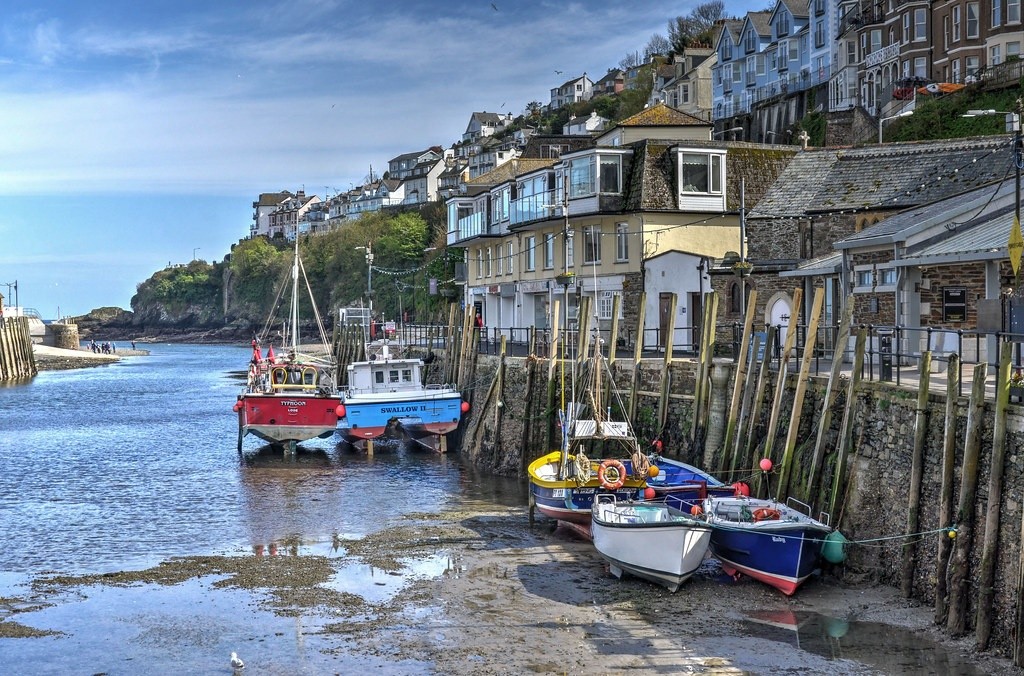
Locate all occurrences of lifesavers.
[598,459,626,490]
[752,508,778,522]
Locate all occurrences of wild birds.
[228,652,244,675]
[554,71,563,75]
[331,103,336,109]
[490,2,498,12]
[500,102,506,108]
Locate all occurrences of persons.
[112,342,116,353]
[475,313,483,353]
[131,340,136,350]
[91,339,111,354]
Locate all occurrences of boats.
[337,310,464,448]
[583,493,713,594]
[624,449,738,519]
[525,312,645,525]
[696,496,833,599]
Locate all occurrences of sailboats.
[235,208,344,460]
